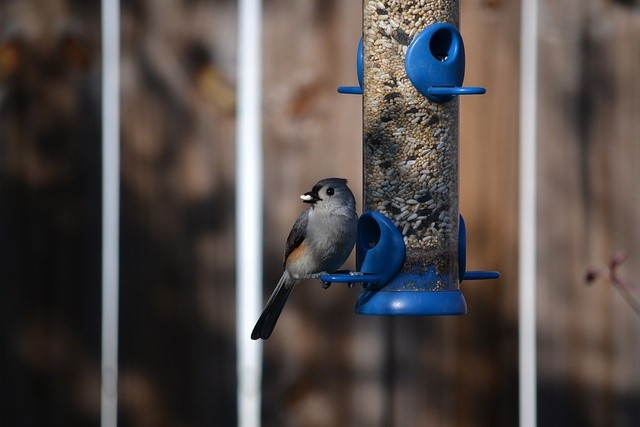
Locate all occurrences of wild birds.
[250,176,360,341]
